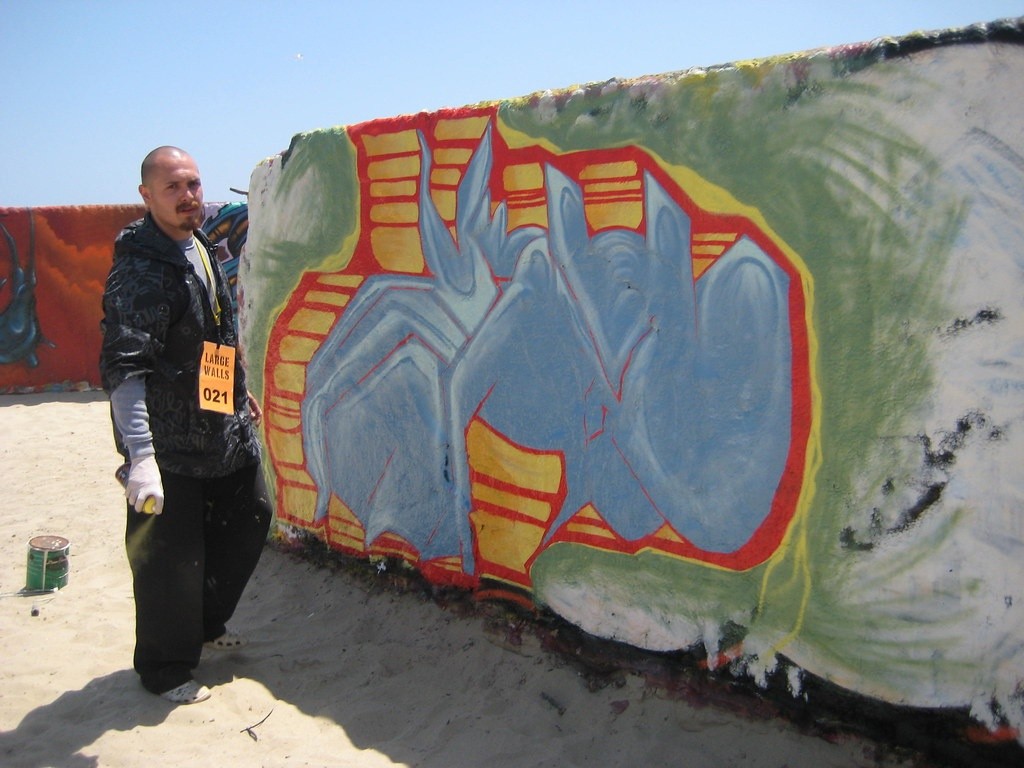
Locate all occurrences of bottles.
[115,462,156,515]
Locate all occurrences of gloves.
[125,454,164,515]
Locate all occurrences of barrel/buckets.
[26,535,70,591]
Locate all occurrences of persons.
[97,145,273,705]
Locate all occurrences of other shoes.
[160,679,211,704]
[202,631,248,651]
